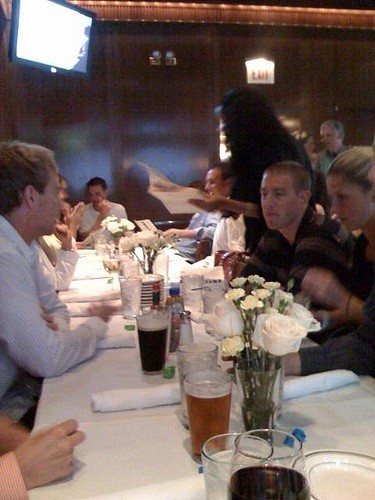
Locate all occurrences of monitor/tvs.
[8,0,97,80]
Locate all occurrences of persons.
[162,86,374,381]
[0,139,117,500]
[77,177,127,249]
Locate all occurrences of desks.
[27,244,375,500]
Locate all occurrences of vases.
[234,360,284,446]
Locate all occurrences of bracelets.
[345,293,352,320]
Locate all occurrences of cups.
[178,342,219,431]
[180,274,228,335]
[119,275,141,320]
[141,275,166,309]
[228,429,310,500]
[134,306,170,375]
[92,233,170,276]
[184,369,233,465]
[201,432,242,499]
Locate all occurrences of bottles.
[179,310,193,347]
[165,286,185,352]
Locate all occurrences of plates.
[306,450,375,500]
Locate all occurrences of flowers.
[214,274,314,441]
[119,231,182,274]
[101,215,135,238]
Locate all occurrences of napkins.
[283,370,360,399]
[89,383,182,412]
[96,335,136,349]
